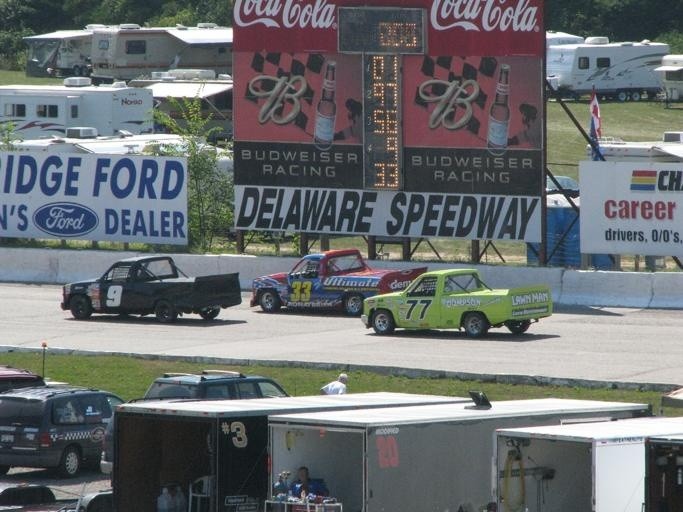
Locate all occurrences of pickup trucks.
[249,245,429,318]
[357,266,554,336]
[58,255,243,325]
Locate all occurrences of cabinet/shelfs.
[264,493,343,512]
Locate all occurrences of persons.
[319,373,350,394]
[287,467,329,498]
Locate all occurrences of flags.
[590,84,605,160]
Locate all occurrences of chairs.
[186,475,216,512]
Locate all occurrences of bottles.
[486,64,512,156]
[314,61,337,151]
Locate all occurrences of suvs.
[1,365,292,481]
